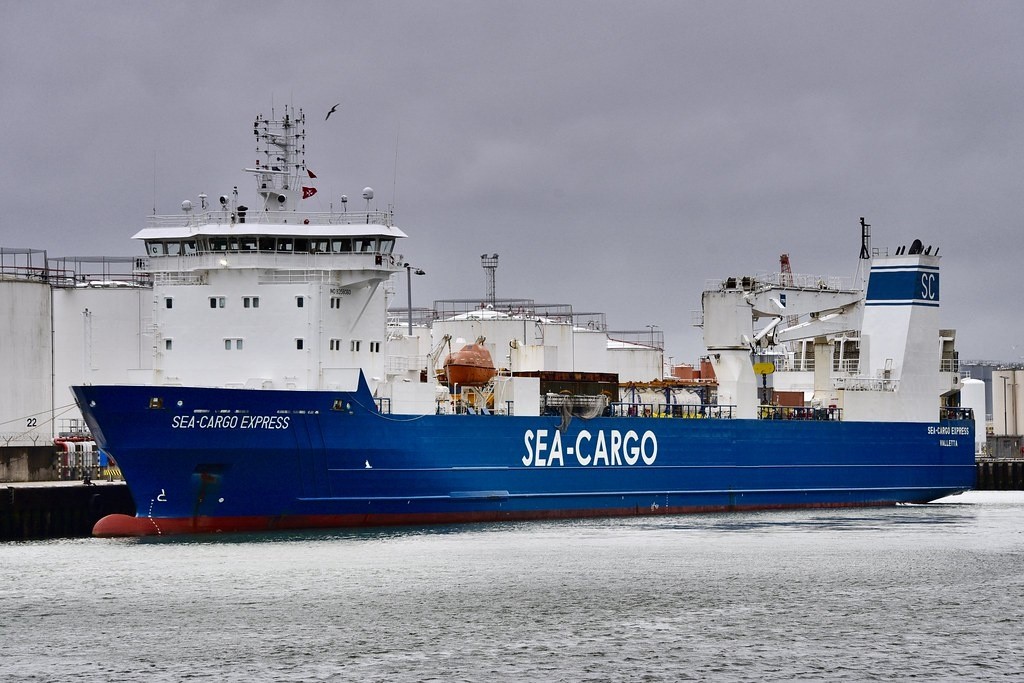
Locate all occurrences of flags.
[302,187,317,199]
[307,169,317,178]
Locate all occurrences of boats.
[442,335,496,388]
[68,90,980,544]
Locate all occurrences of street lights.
[406,266,426,336]
[669,357,674,366]
[1000,375,1009,439]
[646,324,655,348]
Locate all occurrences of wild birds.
[326,103,339,121]
